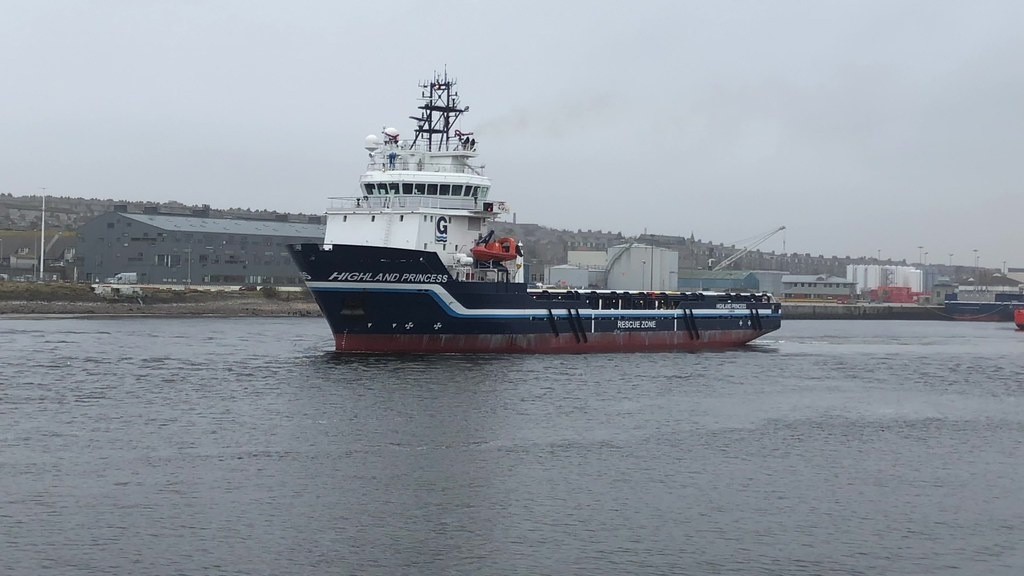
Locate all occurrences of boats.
[470,237,518,262]
[1014,307,1024,331]
[943,287,1024,322]
[286,63,782,356]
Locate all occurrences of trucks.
[107,272,138,284]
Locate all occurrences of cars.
[258,285,277,292]
[238,284,257,292]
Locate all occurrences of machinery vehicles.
[705,225,786,272]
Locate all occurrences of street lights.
[949,253,955,277]
[39,187,46,281]
[1002,260,1006,292]
[649,234,655,291]
[916,245,924,296]
[923,252,929,296]
[972,249,978,302]
